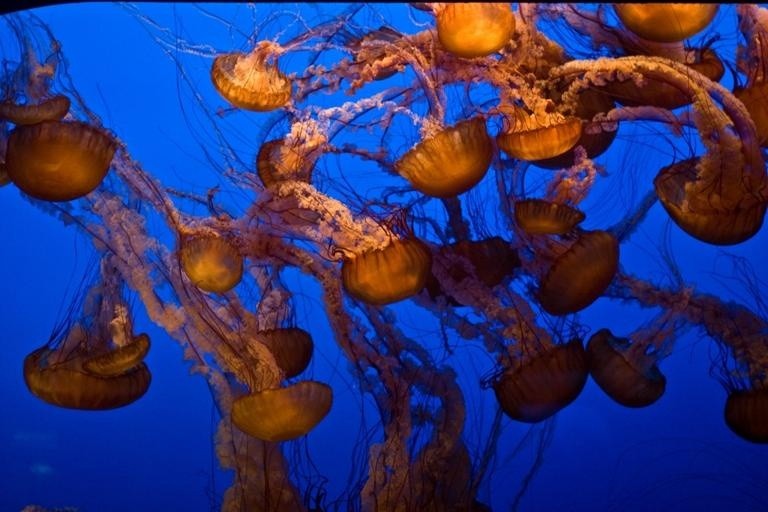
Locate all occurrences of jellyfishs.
[0,0,768,512]
